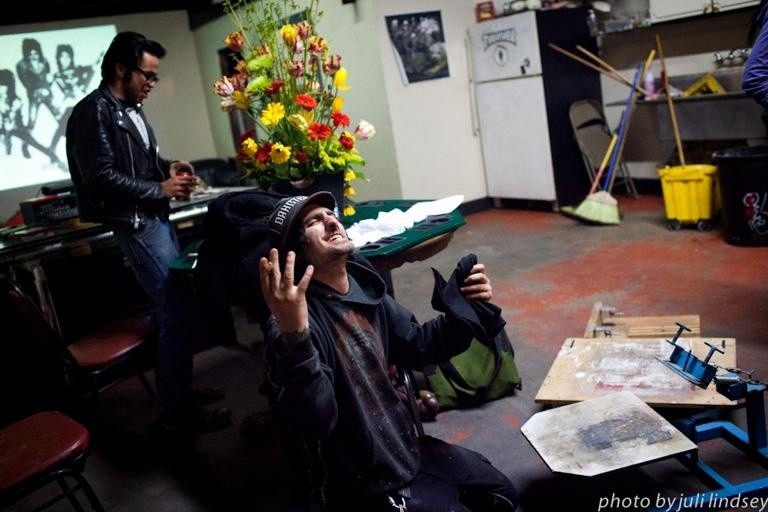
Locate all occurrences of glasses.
[137,67,159,82]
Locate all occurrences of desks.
[167,193,467,435]
[0,186,259,389]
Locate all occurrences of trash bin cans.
[711,145,768,246]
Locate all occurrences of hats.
[265,190,335,252]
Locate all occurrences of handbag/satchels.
[426,337,521,411]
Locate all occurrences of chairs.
[0,274,161,433]
[569,98,639,200]
[0,409,106,512]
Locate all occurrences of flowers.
[211,0,377,217]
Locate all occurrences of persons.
[741,3,768,131]
[257,191,520,512]
[50,45,94,150]
[16,39,64,159]
[0,69,67,174]
[66,31,232,434]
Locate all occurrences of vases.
[271,169,344,215]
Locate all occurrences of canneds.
[175,172,191,202]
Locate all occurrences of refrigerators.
[467,7,607,211]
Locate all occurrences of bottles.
[586,9,599,37]
[642,69,654,100]
[503,2,509,14]
[174,172,195,204]
